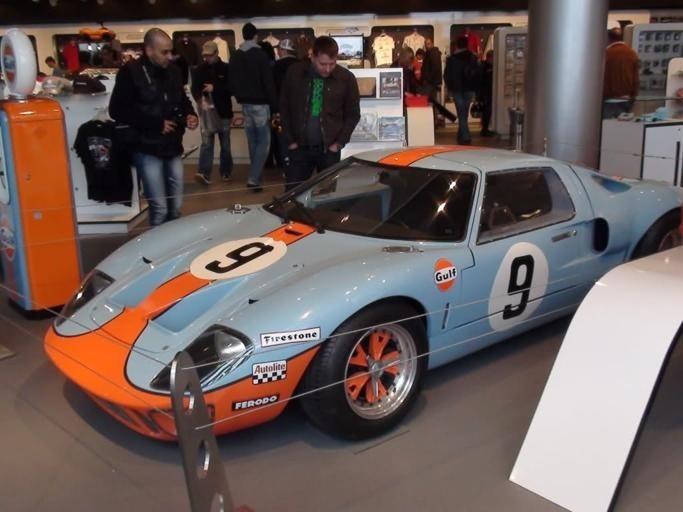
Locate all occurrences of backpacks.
[447,52,485,94]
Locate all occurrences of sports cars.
[37,137,683,457]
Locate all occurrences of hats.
[271,38,298,51]
[201,40,218,55]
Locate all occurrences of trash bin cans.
[508,106,523,150]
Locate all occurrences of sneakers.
[480,129,494,137]
[222,174,232,181]
[194,172,213,185]
[458,138,472,145]
[247,180,263,193]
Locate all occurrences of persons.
[107,29,198,225]
[602,28,639,117]
[37,21,491,194]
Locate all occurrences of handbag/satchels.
[470,103,483,119]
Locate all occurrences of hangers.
[409,28,422,39]
[375,29,390,38]
[211,34,225,43]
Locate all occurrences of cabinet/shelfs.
[488,26,529,139]
[622,22,683,95]
[599,111,683,186]
